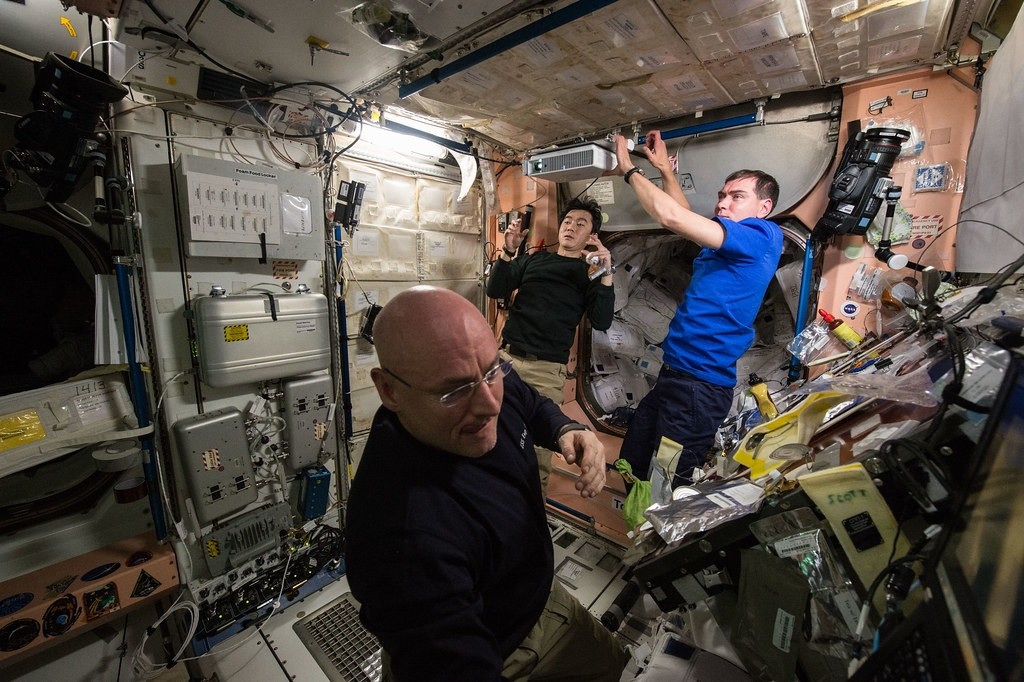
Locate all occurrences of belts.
[501,343,545,360]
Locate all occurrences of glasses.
[381,348,513,409]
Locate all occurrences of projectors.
[528,144,620,183]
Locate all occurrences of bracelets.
[503,246,517,258]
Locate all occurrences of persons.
[486,198,616,505]
[343,285,607,681]
[613,130,783,498]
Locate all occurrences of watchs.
[624,167,646,183]
[557,424,591,439]
